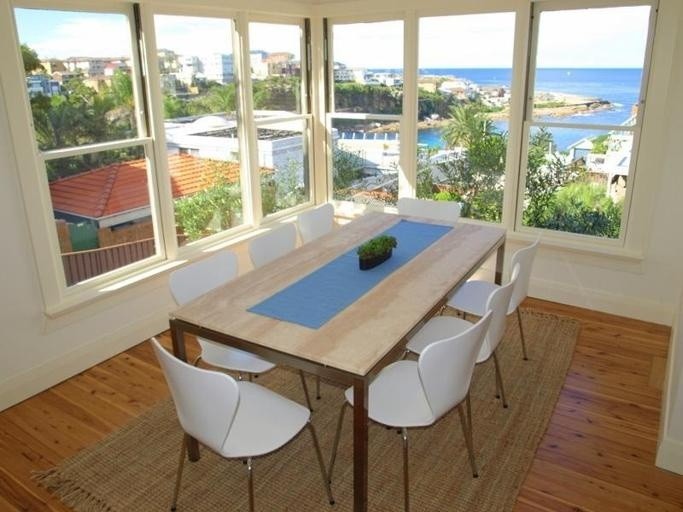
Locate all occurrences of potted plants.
[357,231,398,271]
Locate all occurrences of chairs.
[328,306,494,511]
[396,197,461,227]
[151,336,334,511]
[248,222,297,270]
[167,249,313,416]
[297,202,336,245]
[406,236,542,408]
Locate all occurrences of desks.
[166,210,508,510]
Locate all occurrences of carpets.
[30,308,583,510]
